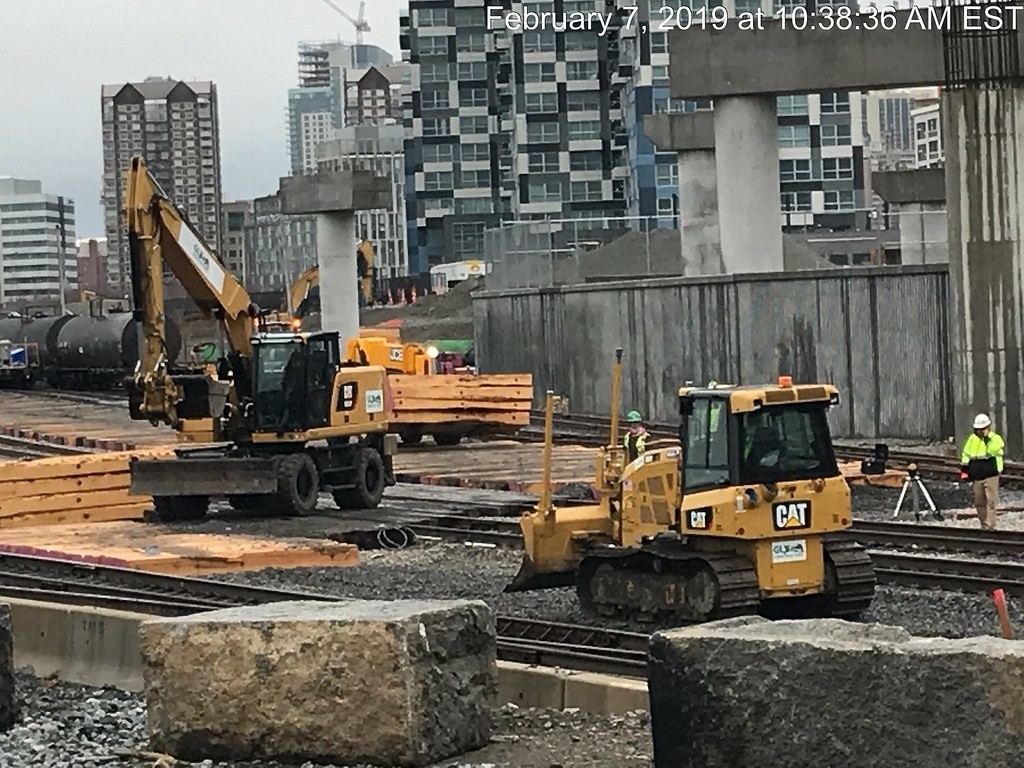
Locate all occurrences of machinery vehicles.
[503,347,877,632]
[343,328,534,448]
[253,239,378,334]
[119,154,398,520]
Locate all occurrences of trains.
[0,294,182,394]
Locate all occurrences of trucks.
[430,260,493,297]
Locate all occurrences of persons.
[743,412,763,461]
[959,413,1005,529]
[622,409,656,464]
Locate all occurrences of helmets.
[626,411,641,422]
[973,414,991,428]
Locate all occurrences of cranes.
[324,0,371,48]
